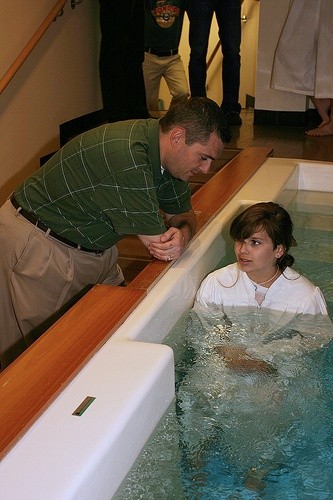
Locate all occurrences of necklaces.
[256,264,280,284]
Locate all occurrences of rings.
[168,256,171,261]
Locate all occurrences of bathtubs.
[0,155,332,496]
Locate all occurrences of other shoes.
[225,112,242,127]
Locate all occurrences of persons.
[268,0,333,137]
[194,200,333,315]
[1,96,233,371]
[142,0,191,119]
[98,0,150,123]
[180,0,246,127]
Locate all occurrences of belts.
[9,193,105,257]
